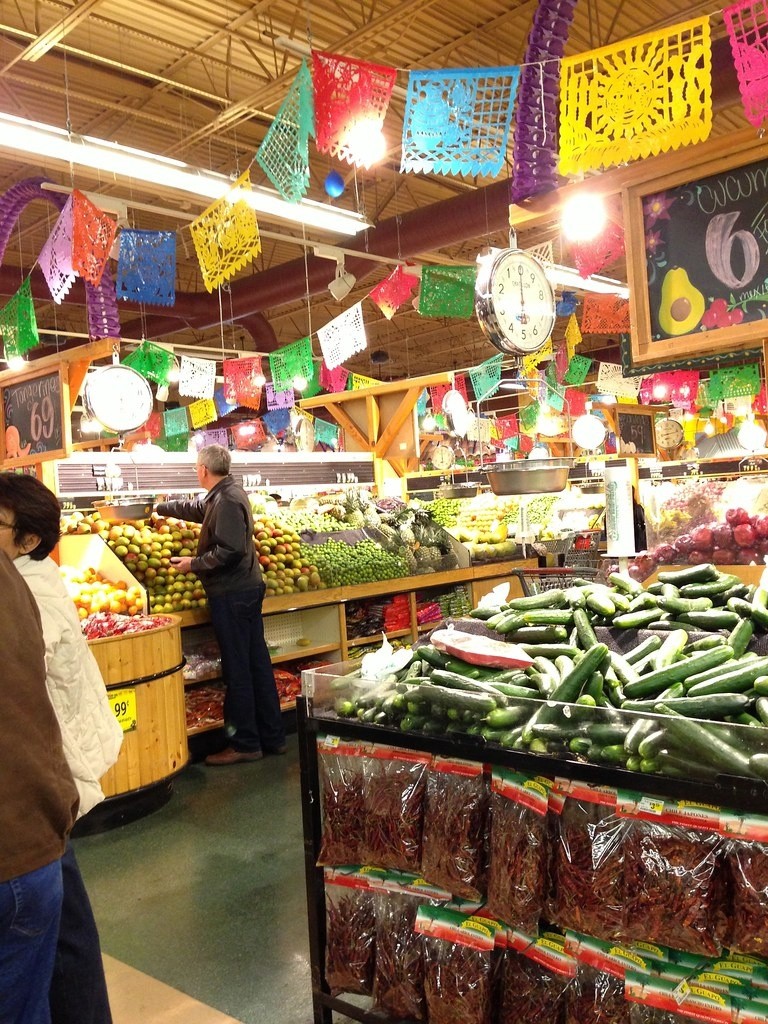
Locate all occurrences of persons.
[152,444,286,765]
[0,549,117,1024]
[0,470,125,1023]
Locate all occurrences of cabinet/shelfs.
[154,555,547,737]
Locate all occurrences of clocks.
[432,445,455,470]
[82,345,153,432]
[654,414,684,450]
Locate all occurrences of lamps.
[475,133,630,299]
[402,265,441,315]
[314,245,356,301]
[0,0,376,236]
[85,194,135,263]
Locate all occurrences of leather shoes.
[270,743,289,755]
[204,747,263,765]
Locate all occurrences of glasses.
[192,464,209,473]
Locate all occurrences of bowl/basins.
[483,457,576,495]
[91,497,158,520]
[437,483,482,499]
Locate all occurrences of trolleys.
[513,529,604,600]
